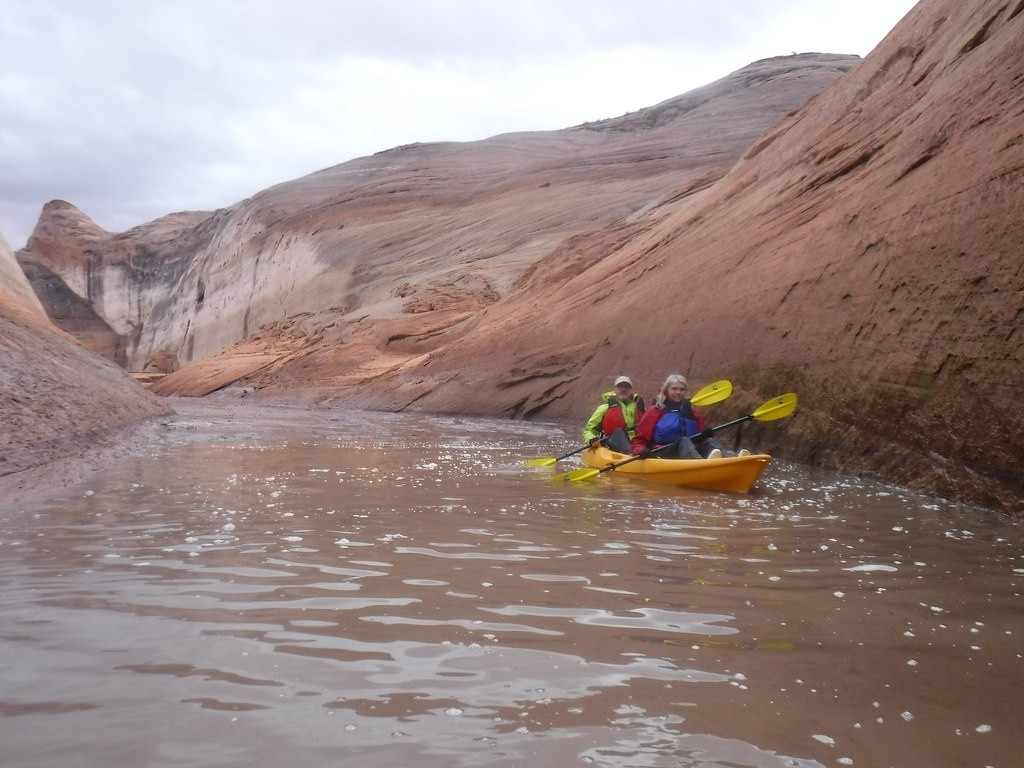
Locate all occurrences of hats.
[614,376,632,387]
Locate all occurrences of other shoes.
[706,449,722,459]
[738,449,750,457]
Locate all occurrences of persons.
[632,374,751,459]
[582,376,645,455]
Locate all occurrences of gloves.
[640,448,657,459]
[702,427,714,439]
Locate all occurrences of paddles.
[551,391,798,483]
[527,380,733,467]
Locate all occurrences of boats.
[582,440,772,493]
